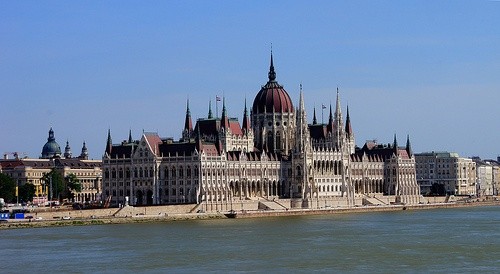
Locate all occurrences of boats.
[224,209,238,219]
